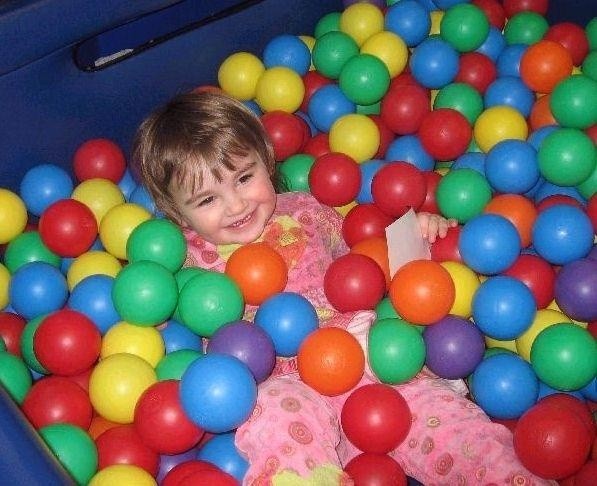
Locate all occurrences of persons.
[131,92,554,486]
